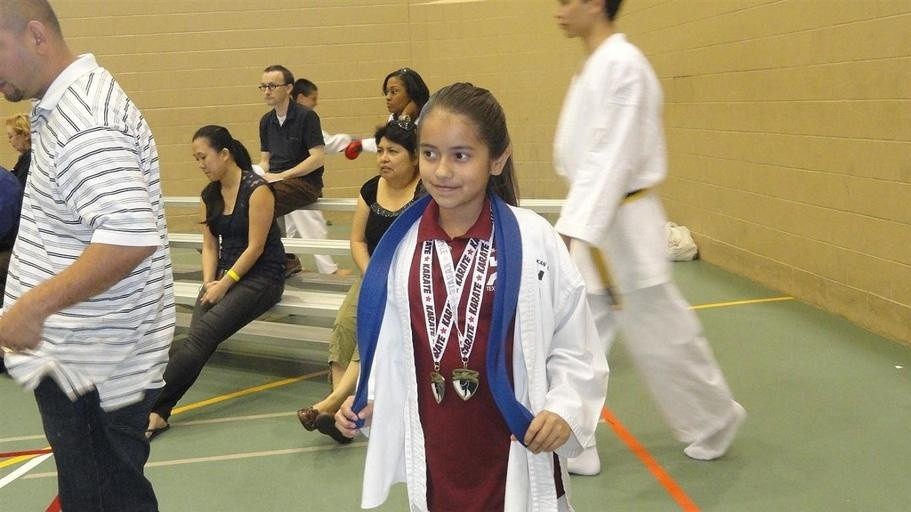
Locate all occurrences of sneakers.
[296,405,320,432]
[315,410,354,444]
[284,253,302,278]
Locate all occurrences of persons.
[142,124,286,440]
[552,0,745,475]
[332,81,610,512]
[346,69,431,160]
[1,166,23,374]
[6,113,31,185]
[283,78,361,275]
[252,64,326,279]
[0,1,175,510]
[298,117,429,443]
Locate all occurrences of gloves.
[345,139,363,160]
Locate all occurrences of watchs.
[399,114,411,122]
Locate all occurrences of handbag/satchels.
[666,220,699,263]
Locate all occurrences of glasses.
[258,84,287,92]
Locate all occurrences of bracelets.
[225,268,241,284]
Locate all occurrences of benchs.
[159,196,358,353]
